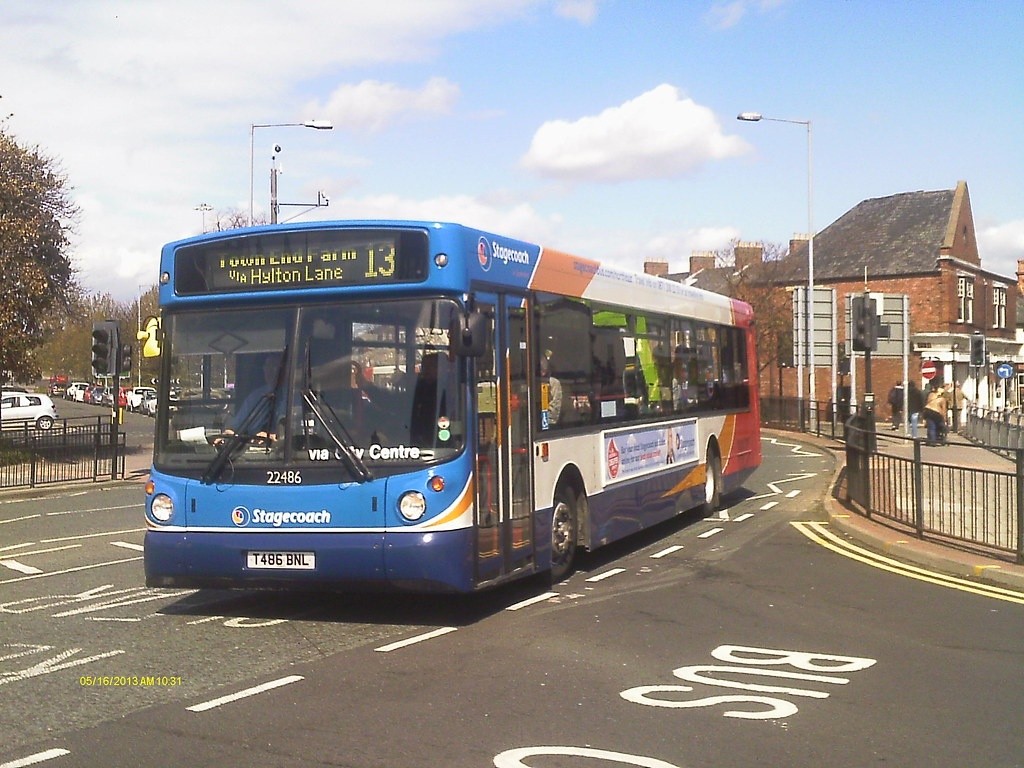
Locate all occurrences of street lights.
[737,112,816,431]
[249,120,334,225]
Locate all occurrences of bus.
[137,220,762,596]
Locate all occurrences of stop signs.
[921,360,936,380]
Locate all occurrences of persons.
[214,353,303,447]
[352,351,452,449]
[887,380,968,447]
[539,354,563,427]
[649,346,690,400]
[119,393,127,408]
[666,426,675,464]
[676,433,681,449]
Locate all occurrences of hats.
[956,380,960,386]
[937,387,945,395]
[265,355,290,374]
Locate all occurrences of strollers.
[925,418,947,445]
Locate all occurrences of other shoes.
[891,425,899,431]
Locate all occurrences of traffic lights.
[838,343,845,357]
[119,344,132,371]
[91,322,116,376]
[969,335,985,365]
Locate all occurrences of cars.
[48,374,89,402]
[1,393,59,430]
[148,393,178,416]
[139,396,157,415]
[83,387,93,403]
[126,387,156,413]
[90,387,105,405]
[2,386,28,393]
[101,387,122,407]
[119,387,133,407]
